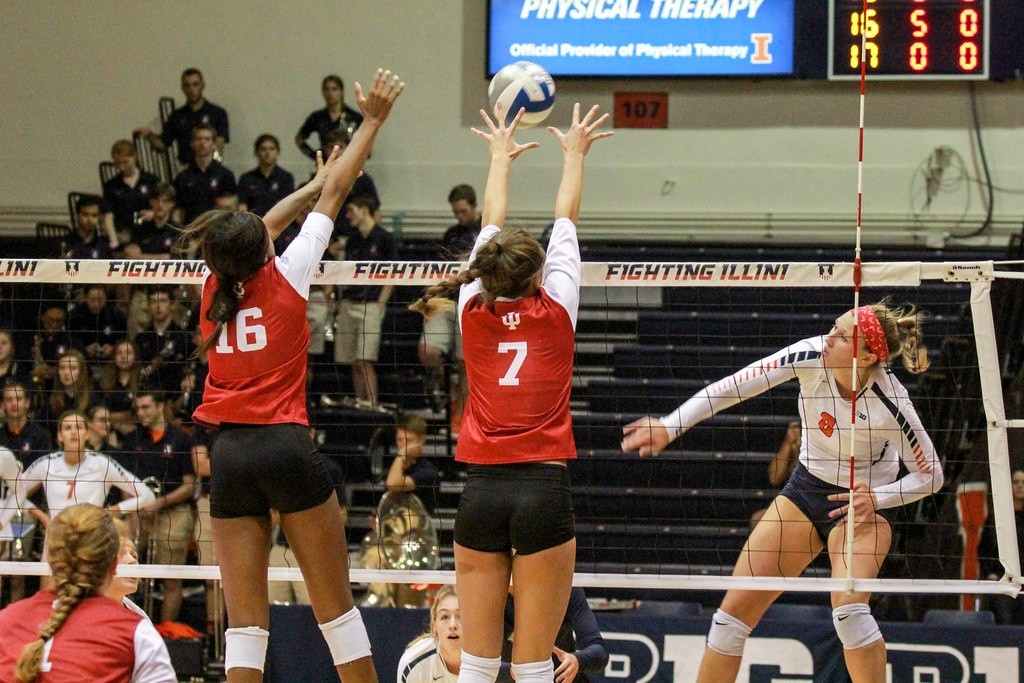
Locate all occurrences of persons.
[397,584,610,683]
[449,100,613,683]
[621,305,943,683]
[747,400,1024,624]
[0,70,484,648]
[0,503,180,683]
[192,68,405,683]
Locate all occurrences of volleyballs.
[487,61,557,131]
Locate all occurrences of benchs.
[0,235,1024,624]
